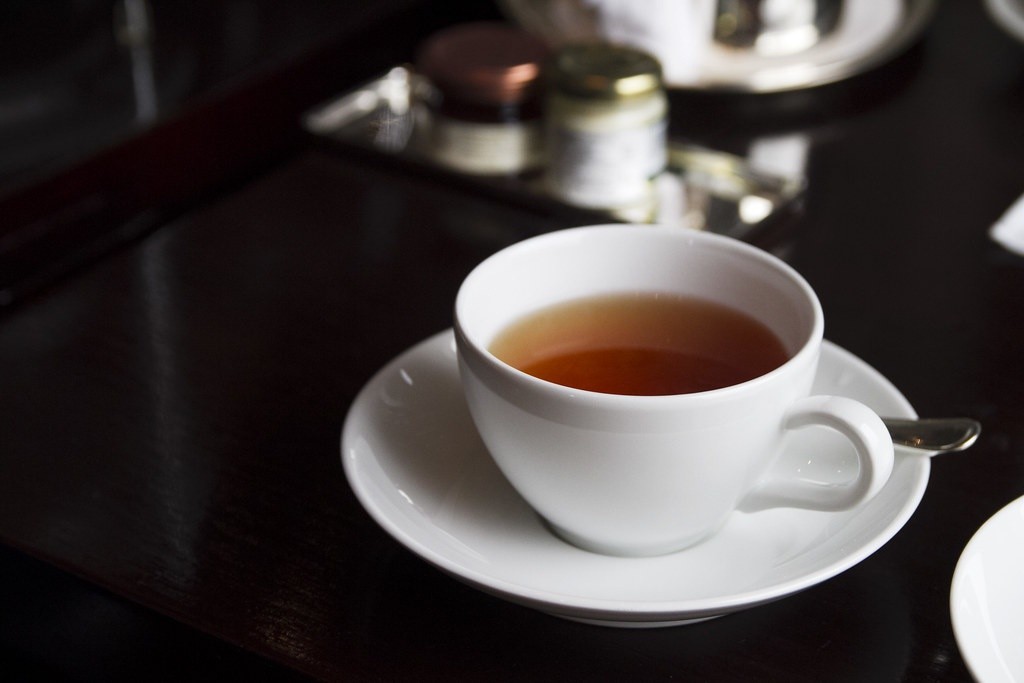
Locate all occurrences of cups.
[454,224,893,555]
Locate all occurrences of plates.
[949,496,1024,683]
[341,330,931,626]
